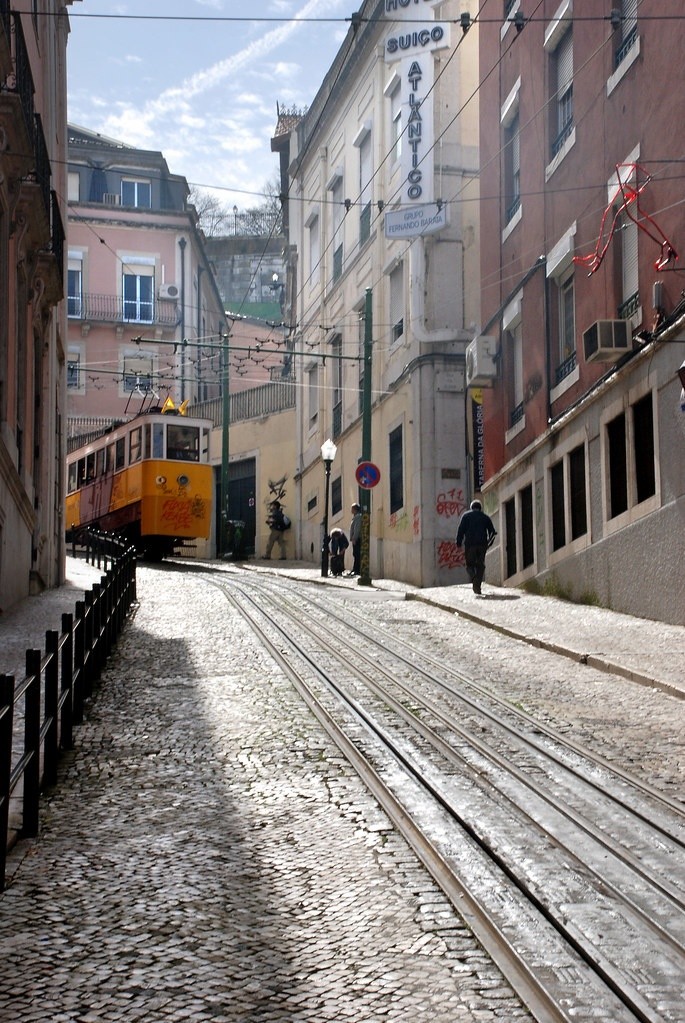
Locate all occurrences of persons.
[261,501,286,560]
[330,528,349,576]
[346,504,362,576]
[456,500,495,594]
[173,442,188,458]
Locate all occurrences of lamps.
[8,172,40,193]
[269,273,284,315]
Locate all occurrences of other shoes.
[278,556,287,560]
[262,555,270,560]
[473,579,482,596]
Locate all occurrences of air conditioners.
[464,336,496,388]
[581,319,632,365]
[158,284,181,299]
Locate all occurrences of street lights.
[320,438,338,577]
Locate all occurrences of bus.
[64,381,215,564]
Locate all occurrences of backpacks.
[280,512,291,529]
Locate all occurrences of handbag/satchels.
[331,556,347,575]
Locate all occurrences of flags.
[161,397,175,415]
[177,401,188,416]
